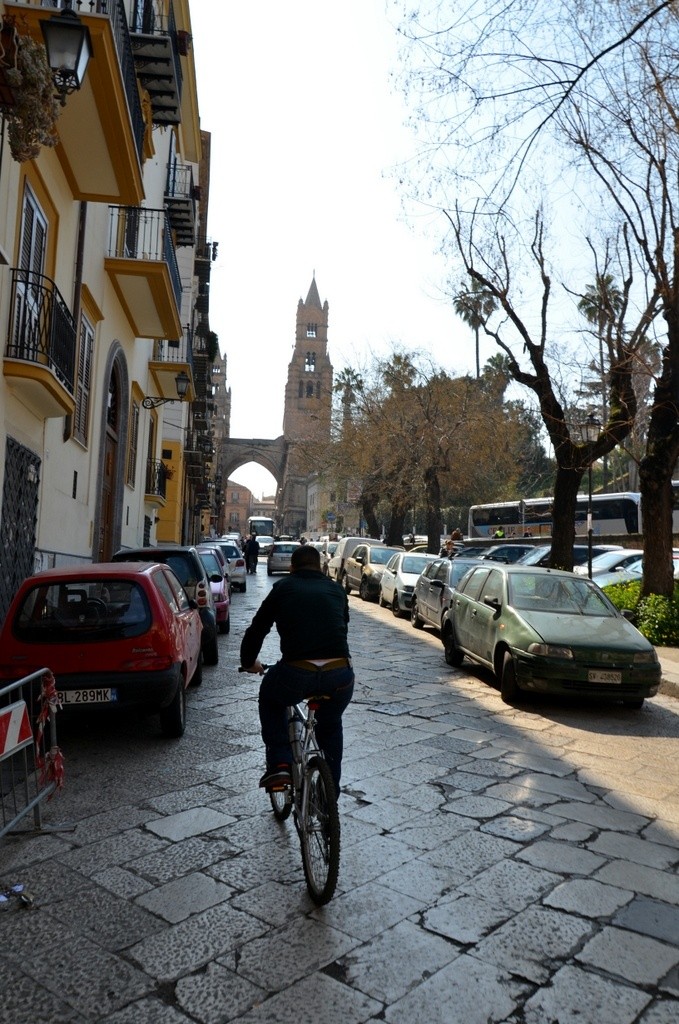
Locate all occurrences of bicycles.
[237,660,341,906]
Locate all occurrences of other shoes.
[258,764,291,788]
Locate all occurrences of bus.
[592,556,679,590]
[467,491,642,540]
[670,480,679,539]
[246,516,276,537]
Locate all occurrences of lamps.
[38,6,95,106]
[142,371,191,409]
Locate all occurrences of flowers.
[194,185,203,201]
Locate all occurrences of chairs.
[53,589,98,619]
[58,589,99,619]
[121,586,145,617]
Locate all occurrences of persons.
[438,540,454,558]
[241,532,259,574]
[408,534,415,543]
[523,527,532,537]
[495,526,505,539]
[451,528,463,541]
[240,545,356,802]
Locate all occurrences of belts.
[289,660,352,671]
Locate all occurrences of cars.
[197,546,231,604]
[110,546,219,666]
[410,557,511,644]
[195,548,230,635]
[202,532,242,551]
[444,564,663,710]
[573,549,643,580]
[255,536,275,554]
[266,535,384,583]
[198,543,246,592]
[408,543,535,562]
[378,551,444,616]
[0,562,204,737]
[514,544,625,572]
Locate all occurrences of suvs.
[340,542,406,602]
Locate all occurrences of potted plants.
[0,21,63,161]
[177,30,193,56]
